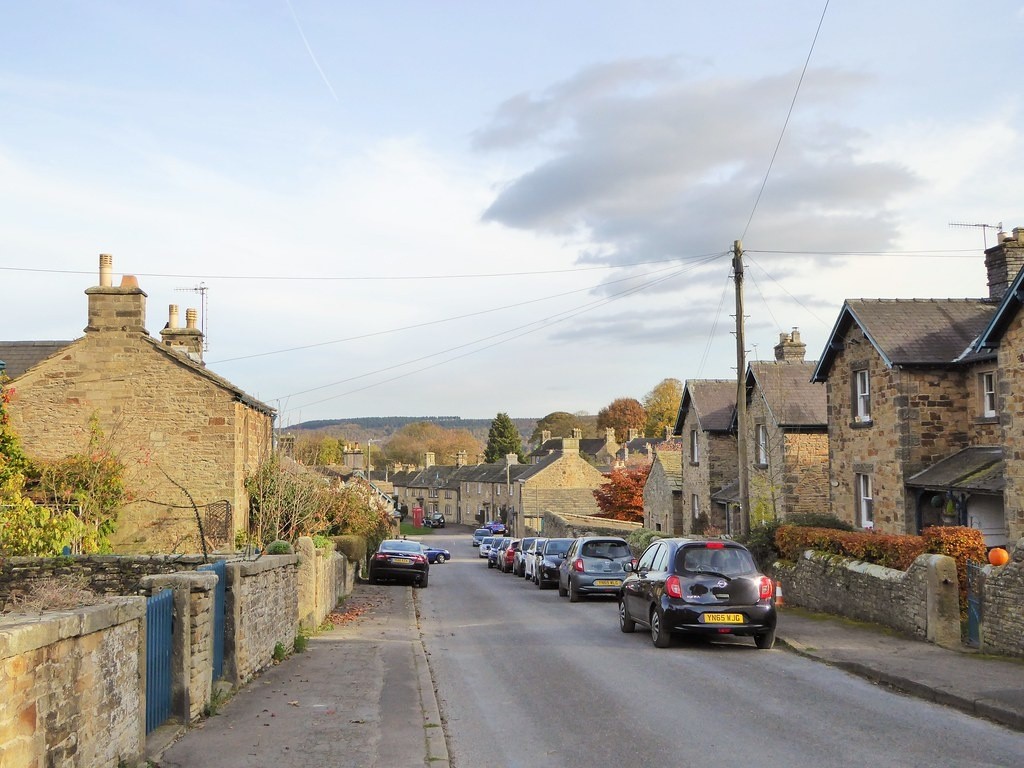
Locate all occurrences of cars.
[395,509,446,528]
[502,539,520,572]
[513,538,537,577]
[478,537,495,558]
[421,544,451,565]
[497,539,510,570]
[488,538,506,568]
[472,528,492,547]
[483,521,505,534]
[535,538,576,590]
[616,536,778,650]
[370,539,429,589]
[558,536,639,601]
[525,538,548,581]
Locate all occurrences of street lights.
[367,439,383,505]
